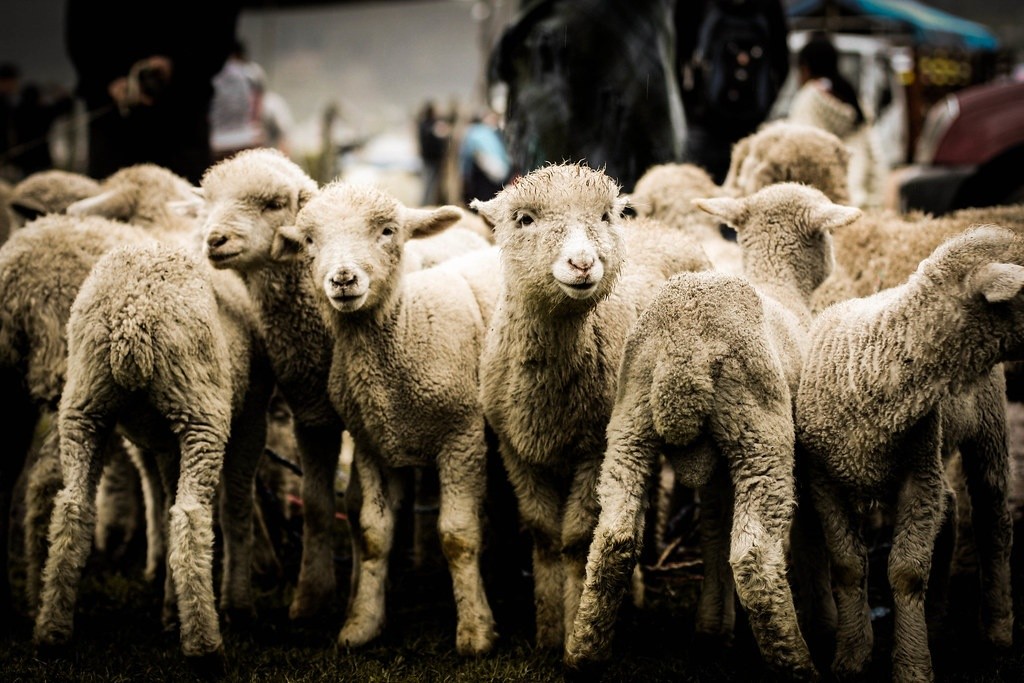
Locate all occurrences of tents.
[786,0,995,164]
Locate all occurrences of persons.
[0,0,1024,217]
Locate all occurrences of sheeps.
[0,119,1024,683]
